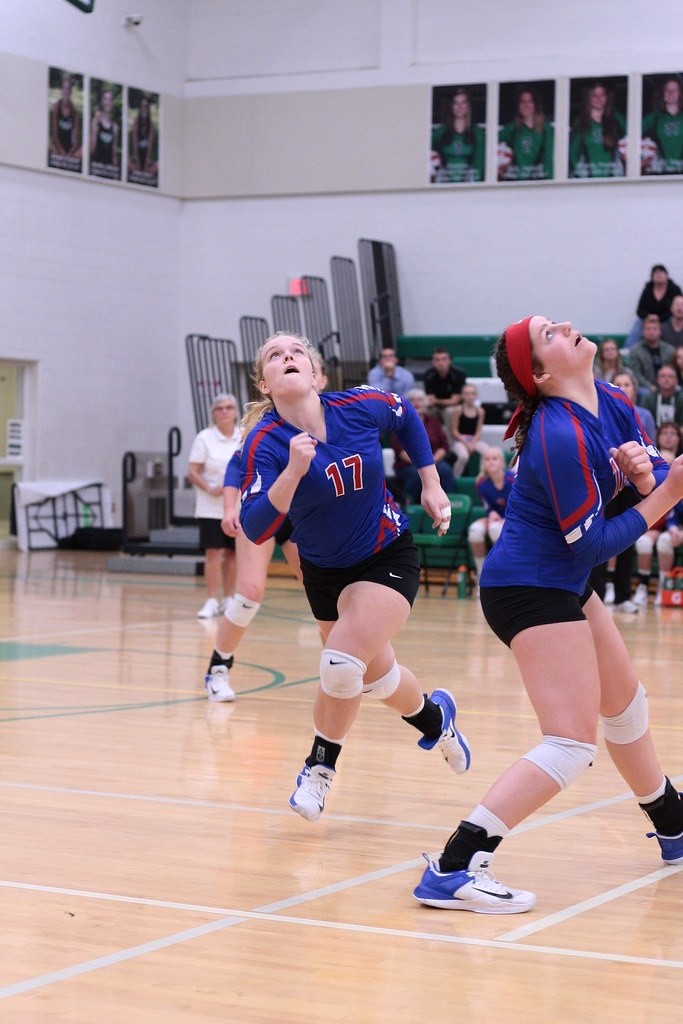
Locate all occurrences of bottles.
[457,565,470,598]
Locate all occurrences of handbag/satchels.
[661,566,683,606]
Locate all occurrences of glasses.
[214,406,236,410]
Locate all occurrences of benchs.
[394,333,682,576]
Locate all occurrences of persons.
[431,87,485,182]
[127,94,158,186]
[187,391,247,617]
[204,423,304,703]
[498,89,553,181]
[570,78,627,177]
[49,72,82,175]
[412,313,683,913]
[364,263,682,618]
[641,73,683,175]
[90,84,121,181]
[240,329,472,824]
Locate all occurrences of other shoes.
[633,584,648,604]
[613,600,638,614]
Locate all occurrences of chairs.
[406,494,472,597]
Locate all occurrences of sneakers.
[413,853,536,914]
[198,598,220,618]
[290,755,336,822]
[418,689,471,773]
[646,825,683,864]
[205,665,235,703]
